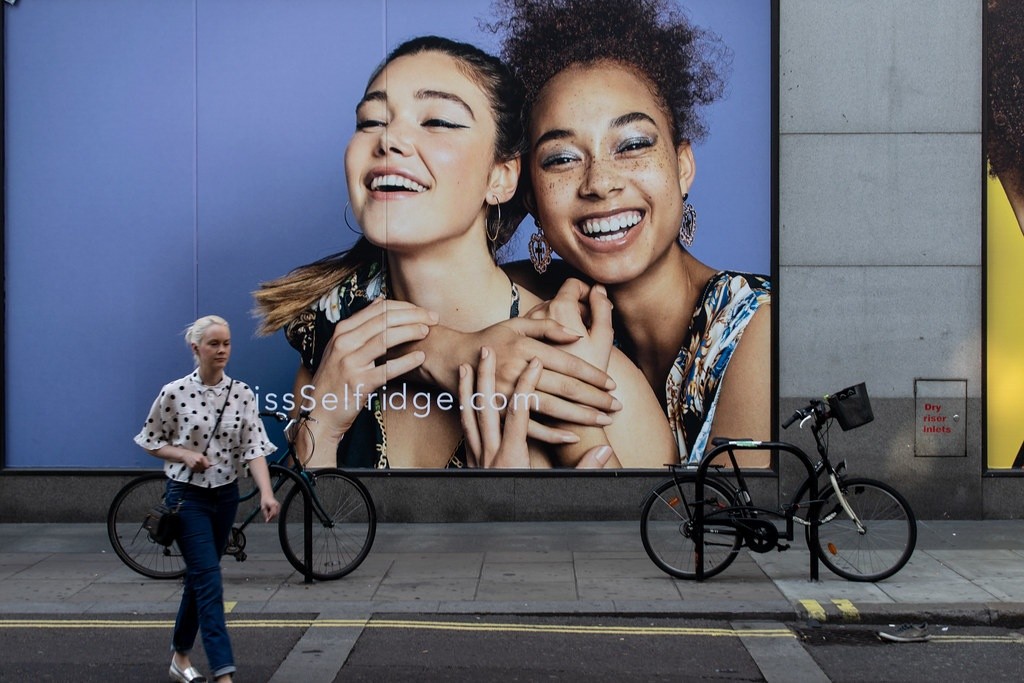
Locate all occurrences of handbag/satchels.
[140,505,177,548]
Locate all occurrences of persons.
[135,313,281,683]
[250,0,771,472]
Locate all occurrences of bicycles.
[107,410,376,581]
[640,381,917,581]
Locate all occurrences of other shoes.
[169,651,206,683]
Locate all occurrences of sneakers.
[877,622,931,642]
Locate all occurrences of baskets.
[828,382,874,432]
